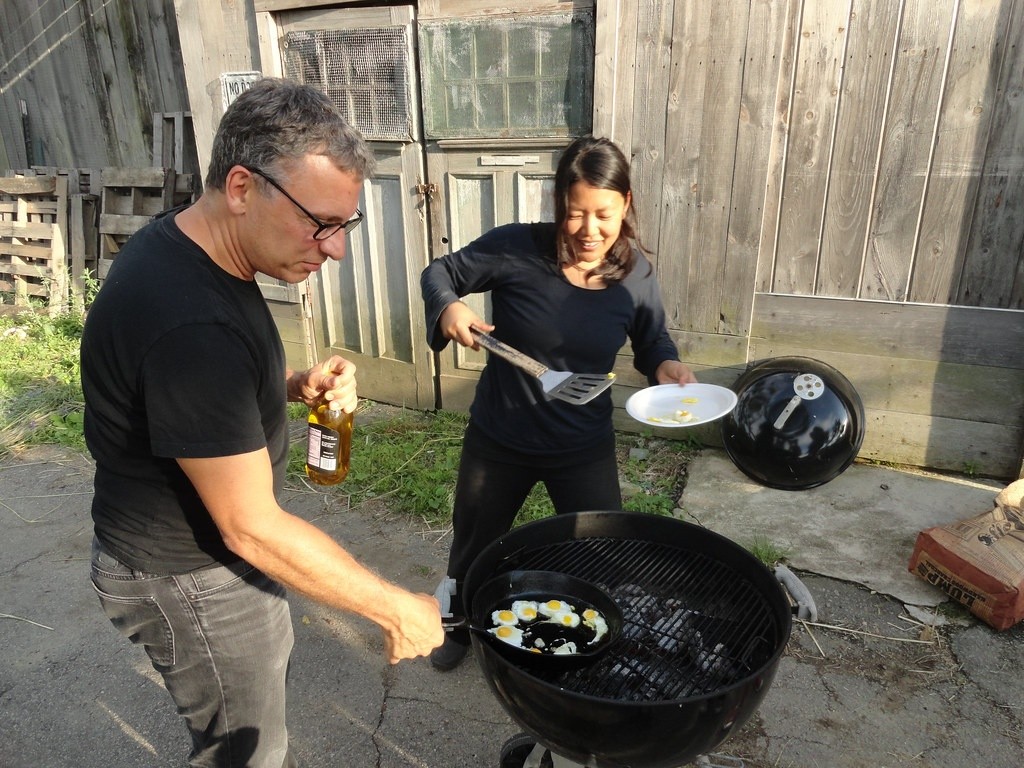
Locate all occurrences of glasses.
[244,165,364,241]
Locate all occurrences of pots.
[439,570,624,663]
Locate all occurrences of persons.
[419,138,698,670]
[79,78,446,768]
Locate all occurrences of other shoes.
[430,630,468,671]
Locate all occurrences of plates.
[626,382,738,426]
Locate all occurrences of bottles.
[305,371,353,484]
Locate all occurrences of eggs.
[488,600,608,656]
[675,409,692,422]
